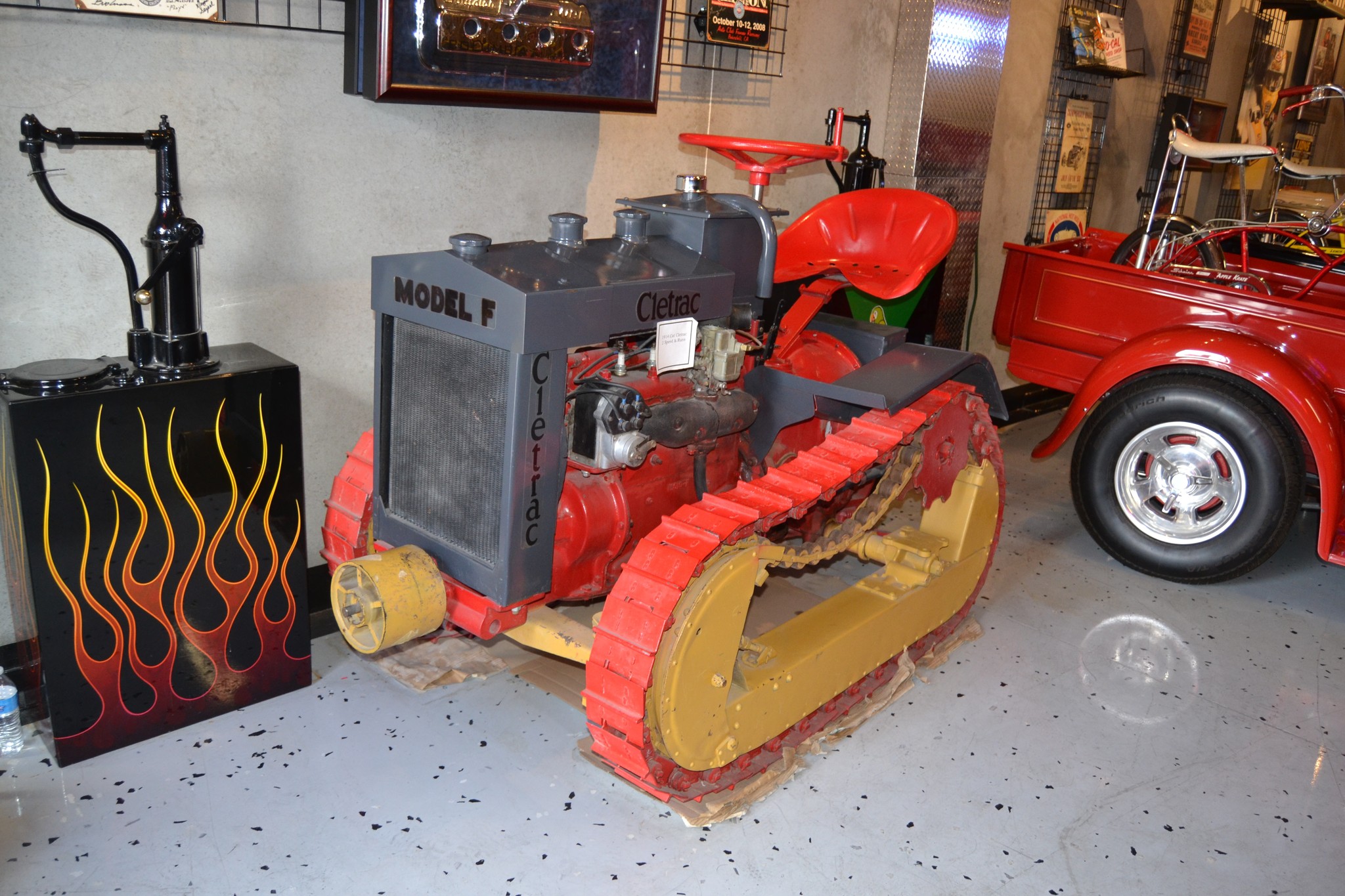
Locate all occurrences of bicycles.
[1109,84,1345,299]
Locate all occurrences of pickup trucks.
[988,221,1344,585]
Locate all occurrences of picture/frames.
[374,0,669,113]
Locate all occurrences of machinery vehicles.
[318,103,1010,802]
